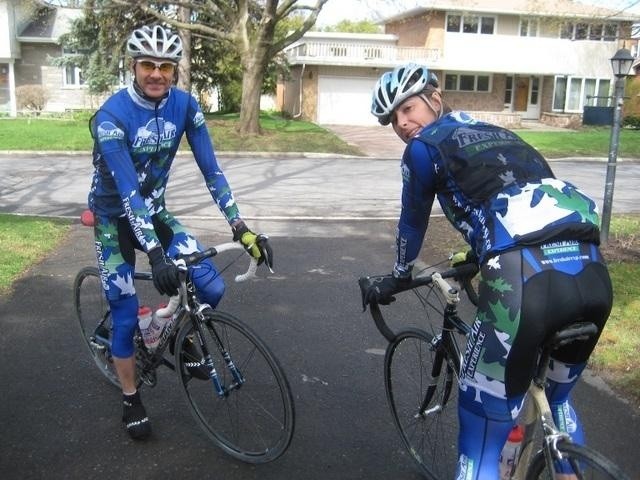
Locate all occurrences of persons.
[361,62,614,480]
[86,24,276,440]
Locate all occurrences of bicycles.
[358,252,632,480]
[73,210,296,464]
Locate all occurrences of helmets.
[126,23,183,62]
[368,62,439,127]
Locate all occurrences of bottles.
[498,425,525,480]
[137,303,169,350]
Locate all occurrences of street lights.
[600,40,636,245]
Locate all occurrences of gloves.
[450,248,481,282]
[147,246,182,297]
[362,268,413,305]
[231,220,274,269]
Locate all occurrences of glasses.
[136,61,176,73]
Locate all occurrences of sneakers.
[121,391,153,441]
[168,333,211,381]
